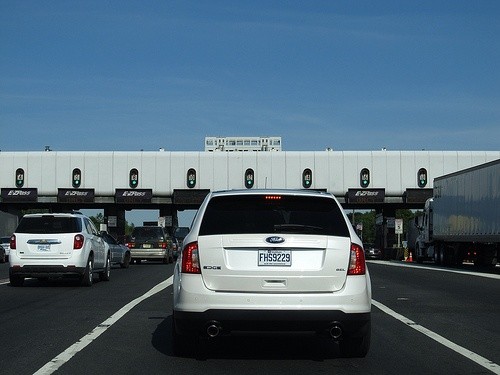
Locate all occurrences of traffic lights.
[417,168,427,187]
[130,168,138,188]
[16,168,24,188]
[360,168,370,188]
[302,168,312,188]
[72,168,81,188]
[187,168,196,188]
[244,168,254,189]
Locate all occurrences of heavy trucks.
[414,159,500,273]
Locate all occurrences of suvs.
[171,188,373,359]
[124,226,190,265]
[9,210,111,287]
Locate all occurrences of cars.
[0,236,11,263]
[362,243,384,260]
[99,231,132,268]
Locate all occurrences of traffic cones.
[407,251,413,263]
